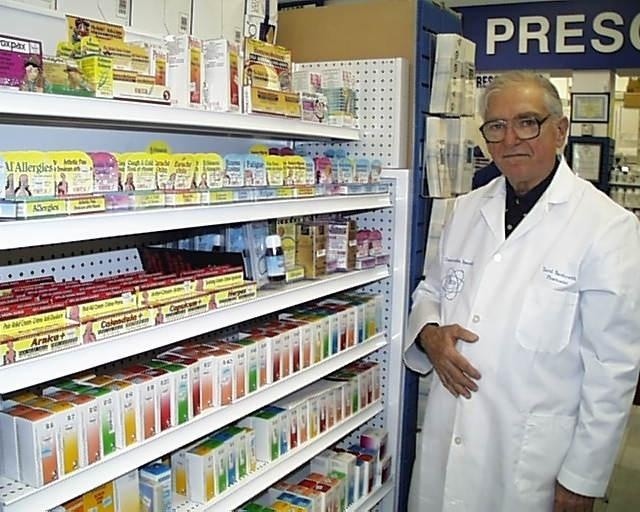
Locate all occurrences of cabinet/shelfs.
[410,24,474,419]
[0,90,410,512]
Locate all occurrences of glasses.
[480,113,550,143]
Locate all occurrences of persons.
[403,68,640,512]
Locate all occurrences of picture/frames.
[571,92,610,123]
